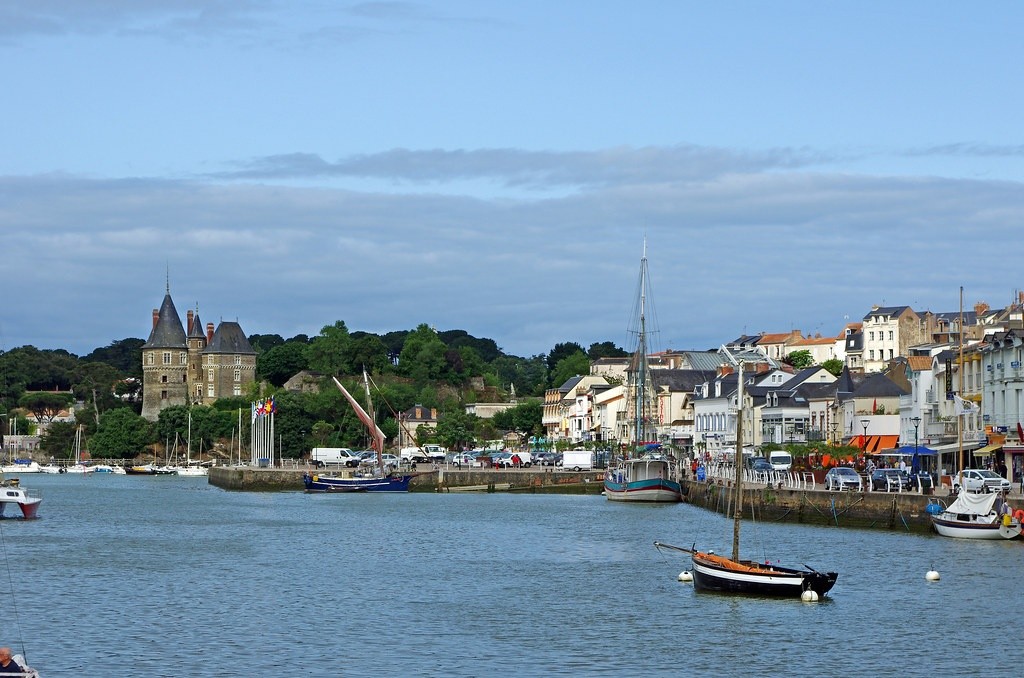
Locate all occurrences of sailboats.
[0,523,40,678]
[925,285,1024,539]
[0,407,247,475]
[604,236,682,503]
[653,359,839,602]
[304,360,440,493]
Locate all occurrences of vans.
[312,448,361,468]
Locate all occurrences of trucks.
[400,443,445,464]
[770,451,792,476]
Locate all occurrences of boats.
[0,478,43,520]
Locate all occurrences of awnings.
[973,444,1005,457]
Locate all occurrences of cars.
[444,451,563,468]
[354,450,401,466]
[752,462,775,480]
[824,467,864,492]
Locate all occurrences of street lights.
[911,417,922,455]
[788,426,795,444]
[829,422,838,450]
[860,419,870,469]
[769,427,775,443]
[805,422,812,444]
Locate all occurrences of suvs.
[952,468,1012,495]
[872,469,913,492]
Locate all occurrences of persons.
[864,459,906,481]
[513,454,520,468]
[999,460,1007,479]
[690,459,698,475]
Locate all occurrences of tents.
[873,445,937,470]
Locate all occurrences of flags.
[252,396,275,425]
[954,395,980,416]
